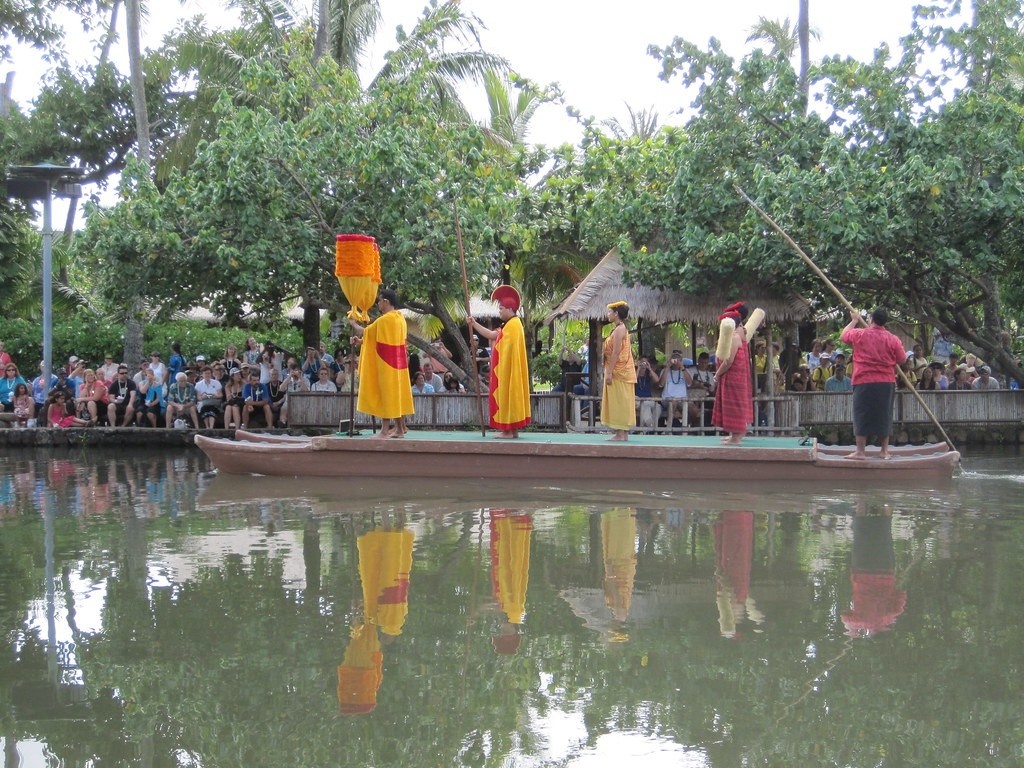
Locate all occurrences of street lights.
[6,162,85,400]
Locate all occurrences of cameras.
[641,361,647,366]
[671,359,678,363]
[207,395,213,399]
[703,382,711,388]
[293,371,299,376]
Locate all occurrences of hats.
[75,360,90,367]
[491,285,520,312]
[140,358,149,369]
[472,335,479,339]
[196,355,206,362]
[69,356,78,362]
[105,354,113,359]
[928,361,945,375]
[819,353,830,358]
[905,351,914,359]
[230,367,242,376]
[834,352,845,359]
[981,366,991,375]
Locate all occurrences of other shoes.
[84,420,93,427]
[277,420,287,428]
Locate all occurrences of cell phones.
[806,369,810,375]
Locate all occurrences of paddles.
[451,197,485,437]
[733,182,963,468]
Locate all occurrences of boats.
[194,429,961,483]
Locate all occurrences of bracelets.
[115,399,117,404]
[62,385,67,389]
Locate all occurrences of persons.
[407,338,589,408]
[490,507,532,656]
[0,337,348,430]
[714,510,753,642]
[329,312,345,342]
[355,502,416,659]
[635,339,1024,436]
[466,285,531,439]
[840,501,907,644]
[348,290,414,439]
[840,308,906,460]
[336,355,360,392]
[710,302,753,445]
[600,507,637,642]
[600,301,637,441]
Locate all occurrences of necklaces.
[310,359,317,372]
[6,377,15,389]
[639,376,646,390]
[177,383,187,402]
[232,382,238,398]
[250,387,259,402]
[759,356,764,364]
[957,382,963,390]
[87,384,94,397]
[249,349,258,362]
[227,357,232,369]
[671,367,680,384]
[118,380,127,394]
[270,382,279,397]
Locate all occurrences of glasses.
[6,369,15,371]
[119,372,127,375]
[153,355,159,357]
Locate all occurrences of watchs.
[682,368,686,371]
[54,386,58,390]
